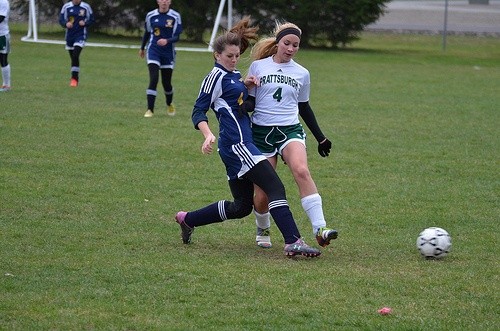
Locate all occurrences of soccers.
[417,225,454,262]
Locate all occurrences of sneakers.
[316,227,338,247]
[175,211,194,244]
[284,235,322,258]
[255,226,272,248]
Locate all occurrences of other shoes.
[168,105,175,115]
[70,79,77,86]
[144,109,154,117]
[0,86,11,92]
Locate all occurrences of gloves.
[238,101,252,118]
[318,139,331,157]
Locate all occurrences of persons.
[174,14,321,258]
[57,0,95,87]
[0,0,11,91]
[139,0,182,117]
[238,18,338,248]
